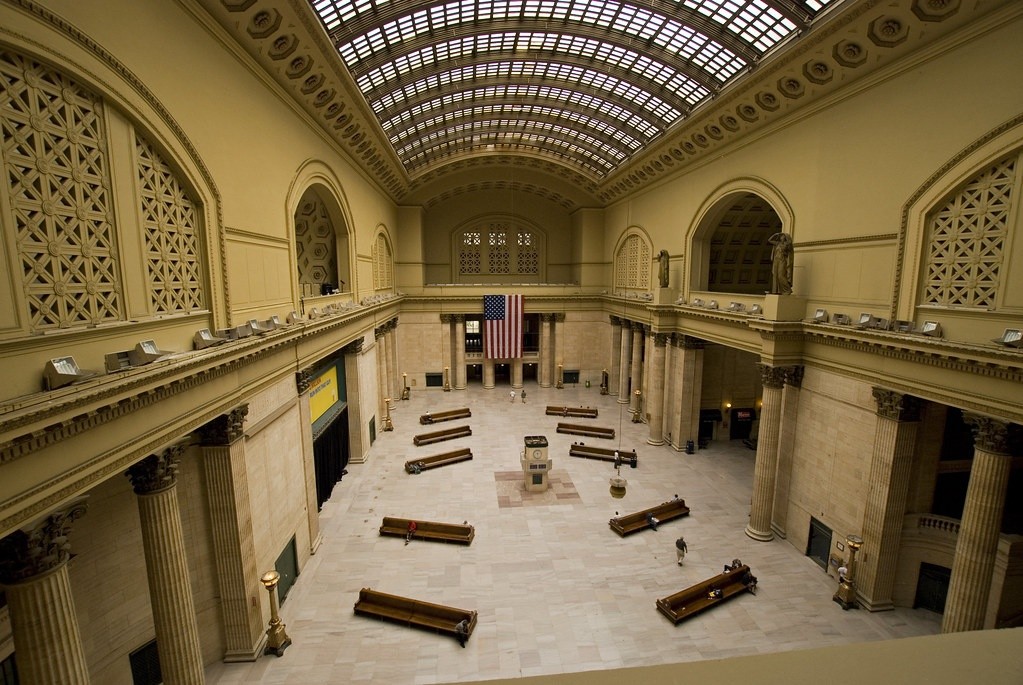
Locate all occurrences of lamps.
[402,372,410,400]
[814,308,940,338]
[601,290,653,301]
[193,291,397,351]
[991,328,1023,348]
[42,356,83,389]
[673,297,761,315]
[556,364,564,389]
[383,397,393,431]
[726,402,732,412]
[441,366,453,392]
[259,571,292,658]
[136,340,175,365]
[631,389,642,423]
[832,535,863,611]
[600,370,608,395]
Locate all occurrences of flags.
[483,295,524,359]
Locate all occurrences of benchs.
[420,408,471,425]
[405,448,473,475]
[608,497,690,540]
[414,425,473,447]
[353,587,478,642]
[379,518,475,547]
[569,444,637,466]
[556,423,615,440]
[546,406,598,418]
[656,558,757,626]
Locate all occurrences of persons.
[646,511,657,531]
[464,520,474,528]
[520,390,526,404]
[405,521,416,544]
[562,405,680,518]
[767,232,794,294]
[676,536,687,566]
[837,565,848,581]
[657,250,670,287]
[723,558,742,572]
[456,620,468,648]
[509,390,516,403]
[743,572,756,595]
[425,411,433,424]
